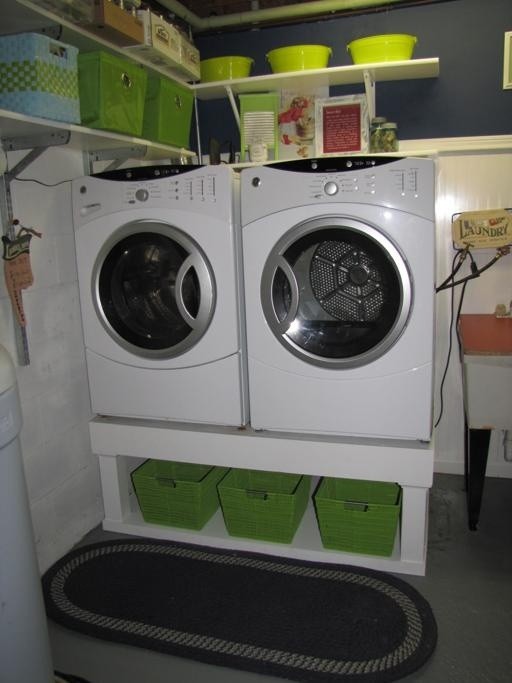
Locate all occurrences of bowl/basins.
[199,56,256,81]
[344,35,419,70]
[263,43,334,73]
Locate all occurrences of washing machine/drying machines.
[69,162,248,434]
[238,151,438,446]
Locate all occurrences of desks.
[454,312,512,534]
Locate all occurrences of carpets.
[41,538,439,682]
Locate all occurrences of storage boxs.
[131,458,230,532]
[122,9,201,82]
[1,33,82,124]
[313,477,402,558]
[81,52,149,138]
[142,78,196,150]
[35,1,94,26]
[215,467,310,545]
[80,1,145,48]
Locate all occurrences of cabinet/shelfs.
[1,0,442,170]
